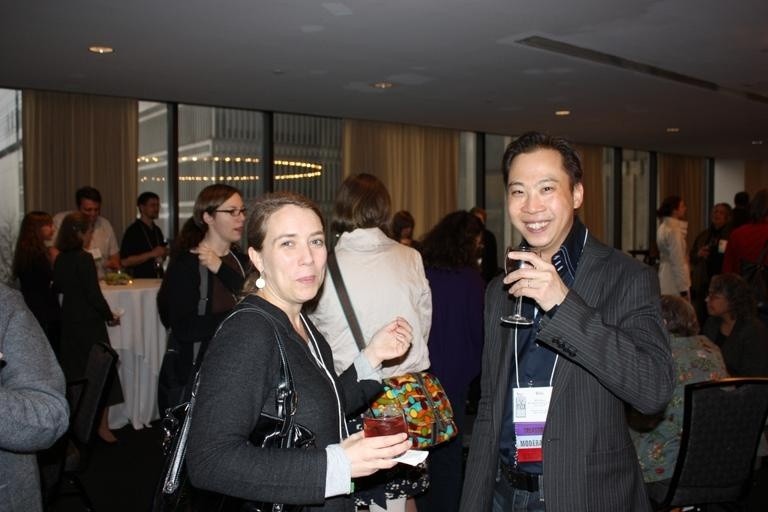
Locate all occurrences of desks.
[627,249,649,258]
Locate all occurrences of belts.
[500,462,543,492]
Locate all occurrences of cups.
[718,240,727,253]
[363,408,410,459]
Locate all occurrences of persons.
[0,268,71,512]
[393,207,498,451]
[655,189,767,325]
[44,186,121,276]
[701,274,766,387]
[312,172,432,511]
[186,192,412,512]
[120,193,169,278]
[156,184,252,429]
[55,212,125,449]
[459,130,671,510]
[12,211,73,326]
[633,294,730,503]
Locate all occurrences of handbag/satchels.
[362,372,457,451]
[157,340,203,411]
[154,401,316,511]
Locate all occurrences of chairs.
[35,333,119,512]
[653,375,767,512]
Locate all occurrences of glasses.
[213,208,247,216]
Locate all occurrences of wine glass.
[153,262,162,280]
[500,246,542,325]
